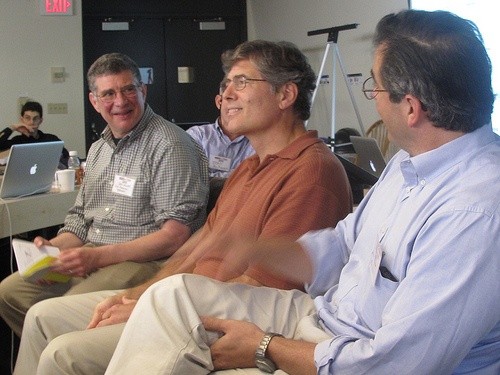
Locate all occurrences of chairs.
[367,120,389,158]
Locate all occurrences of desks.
[0,173,78,240]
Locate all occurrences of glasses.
[93,85,142,103]
[362,76,427,112]
[219,75,270,95]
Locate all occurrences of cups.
[57,170,75,193]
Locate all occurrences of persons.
[0,53,209,338]
[11,38,353,375]
[186,75,256,215]
[104,10,500,375]
[0,100,71,169]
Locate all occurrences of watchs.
[255,332,285,374]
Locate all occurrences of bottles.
[68,150,80,174]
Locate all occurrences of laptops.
[349,135,387,178]
[0,141,64,199]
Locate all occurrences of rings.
[69,270,72,272]
